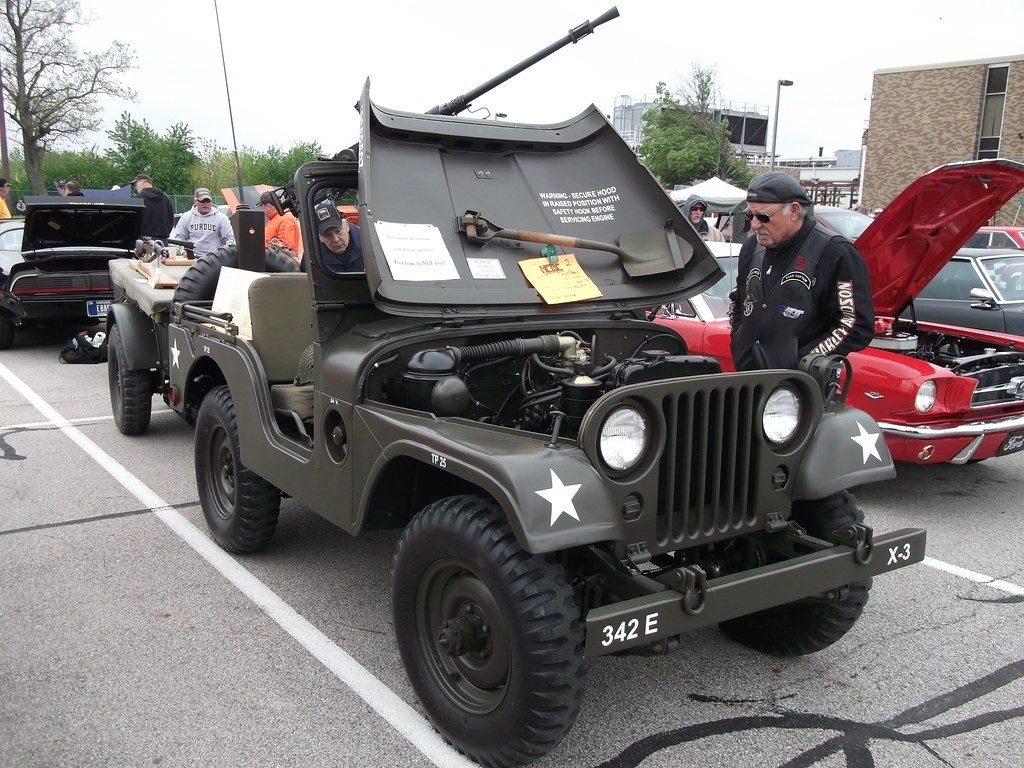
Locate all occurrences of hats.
[0,178,12,187]
[747,172,812,204]
[315,204,343,235]
[130,174,151,186]
[194,188,212,201]
[262,191,282,206]
[57,180,67,187]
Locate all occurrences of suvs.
[646,158,1024,464]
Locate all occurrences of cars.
[812,205,877,244]
[898,248,1024,338]
[960,226,1024,293]
[0,196,147,349]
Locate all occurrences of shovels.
[464,213,694,279]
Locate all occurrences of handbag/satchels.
[59,337,108,364]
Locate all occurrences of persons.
[16,200,26,215]
[256,191,300,257]
[131,174,174,248]
[299,204,366,275]
[685,194,725,242]
[0,178,12,219]
[728,172,874,369]
[65,180,84,196]
[58,180,67,197]
[174,188,236,257]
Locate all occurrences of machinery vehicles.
[107,4,928,768]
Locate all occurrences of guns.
[270,5,620,219]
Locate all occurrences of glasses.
[197,199,211,204]
[743,207,783,223]
[691,206,705,211]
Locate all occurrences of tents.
[670,176,749,215]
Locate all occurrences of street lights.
[770,79,793,172]
